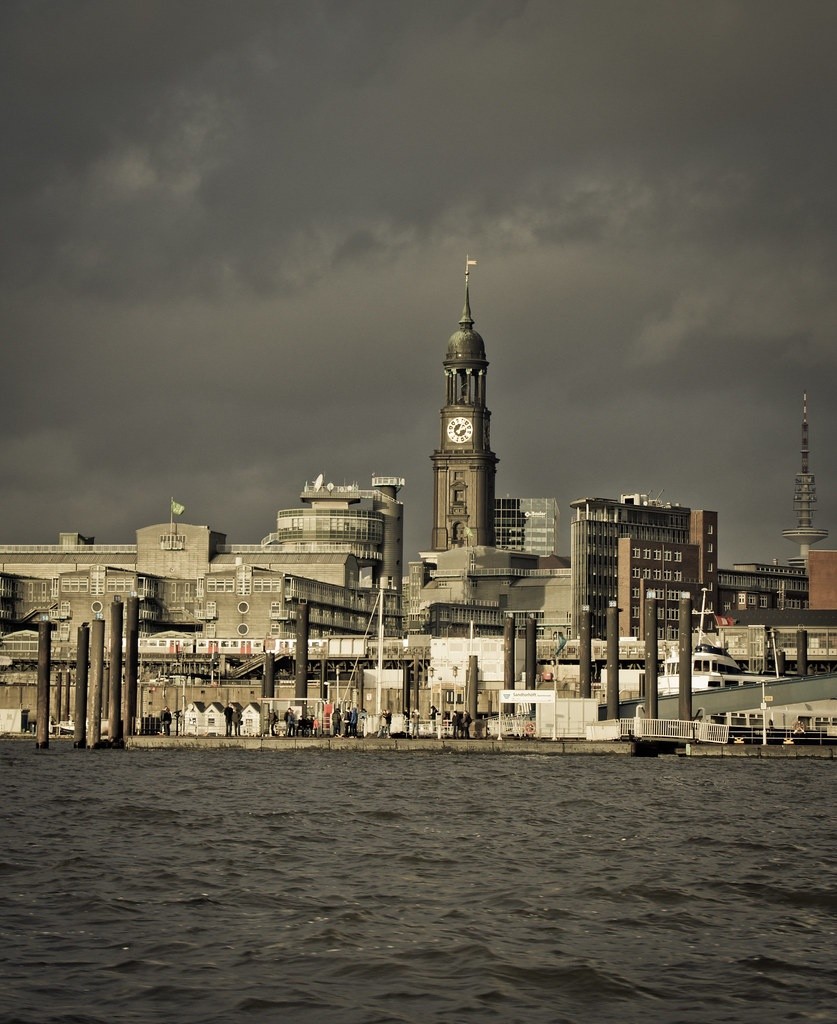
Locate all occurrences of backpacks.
[466,714,472,724]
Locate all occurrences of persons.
[284,707,319,738]
[452,711,462,739]
[160,706,168,735]
[224,703,233,737]
[333,706,358,739]
[462,711,471,740]
[377,710,392,738]
[269,709,277,735]
[359,708,368,720]
[232,707,242,736]
[429,706,438,720]
[164,708,171,736]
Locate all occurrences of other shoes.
[349,736,354,738]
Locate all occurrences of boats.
[658,587,837,744]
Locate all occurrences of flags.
[553,636,567,654]
[170,499,186,515]
[465,526,475,538]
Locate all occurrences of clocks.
[446,417,474,443]
